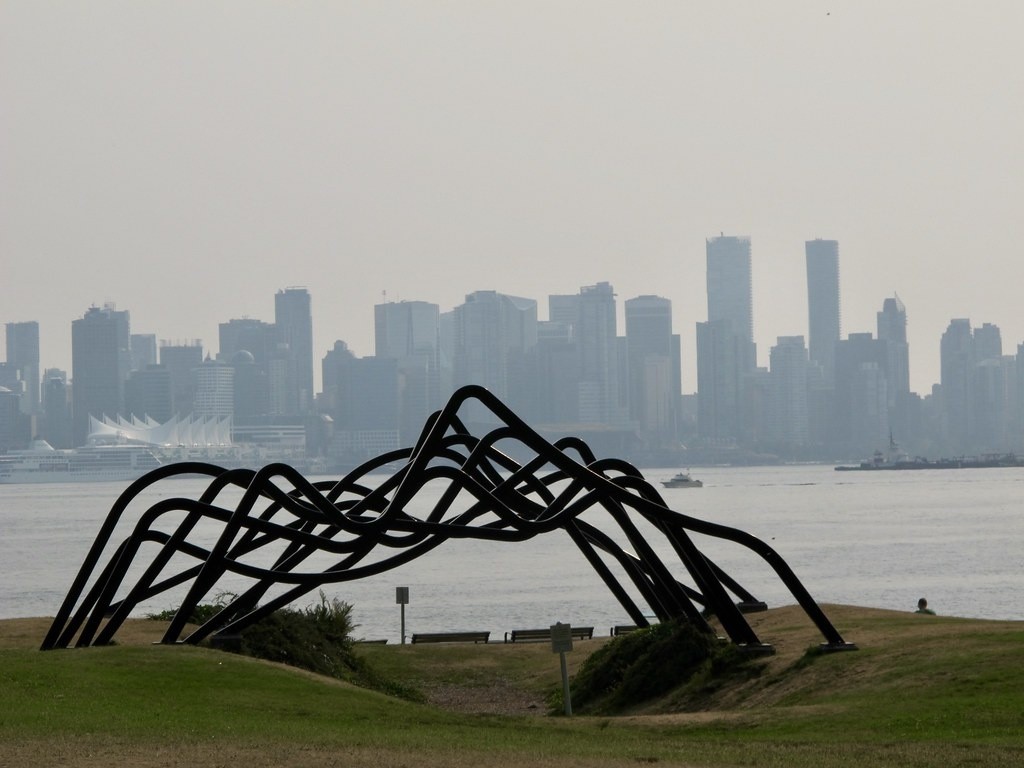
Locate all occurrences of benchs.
[611,625,640,638]
[504,627,595,644]
[411,631,491,643]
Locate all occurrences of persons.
[915,598,936,614]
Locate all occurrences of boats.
[833,437,1024,469]
[662,472,702,489]
[0,439,162,483]
